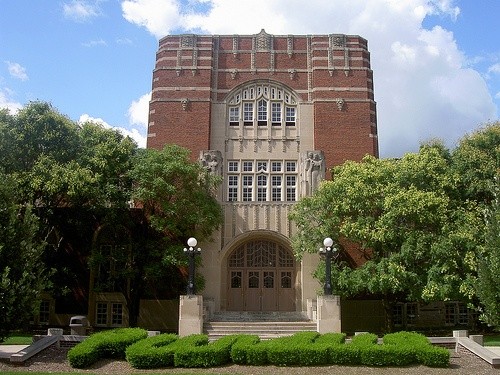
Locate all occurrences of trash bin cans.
[68,315,89,335]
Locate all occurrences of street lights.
[183,237,201,296]
[319,238,337,295]
